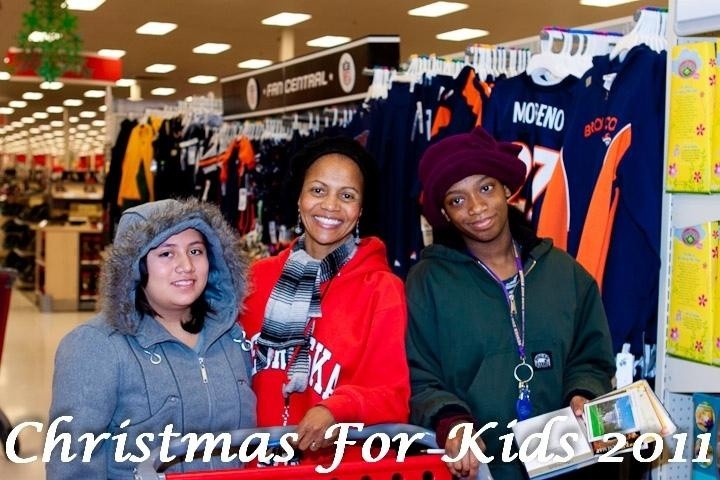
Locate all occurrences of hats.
[418,126,527,229]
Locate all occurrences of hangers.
[127,8,668,147]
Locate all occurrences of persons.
[40,195,260,480]
[404,123,620,480]
[236,134,413,469]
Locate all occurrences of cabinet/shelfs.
[0,192,103,312]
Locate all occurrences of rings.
[311,441,317,449]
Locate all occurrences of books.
[511,379,679,480]
